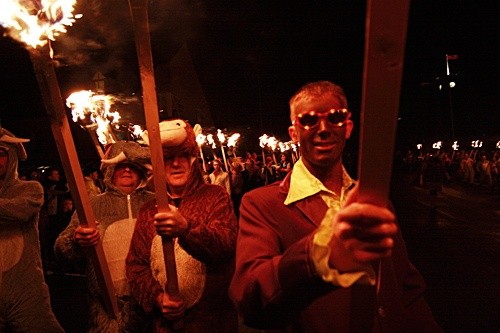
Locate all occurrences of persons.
[197,154,290,195]
[124,119,237,333]
[19,169,101,275]
[228,82,439,333]
[407,150,500,188]
[0,128,65,333]
[55,140,148,333]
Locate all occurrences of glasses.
[295,108,349,131]
